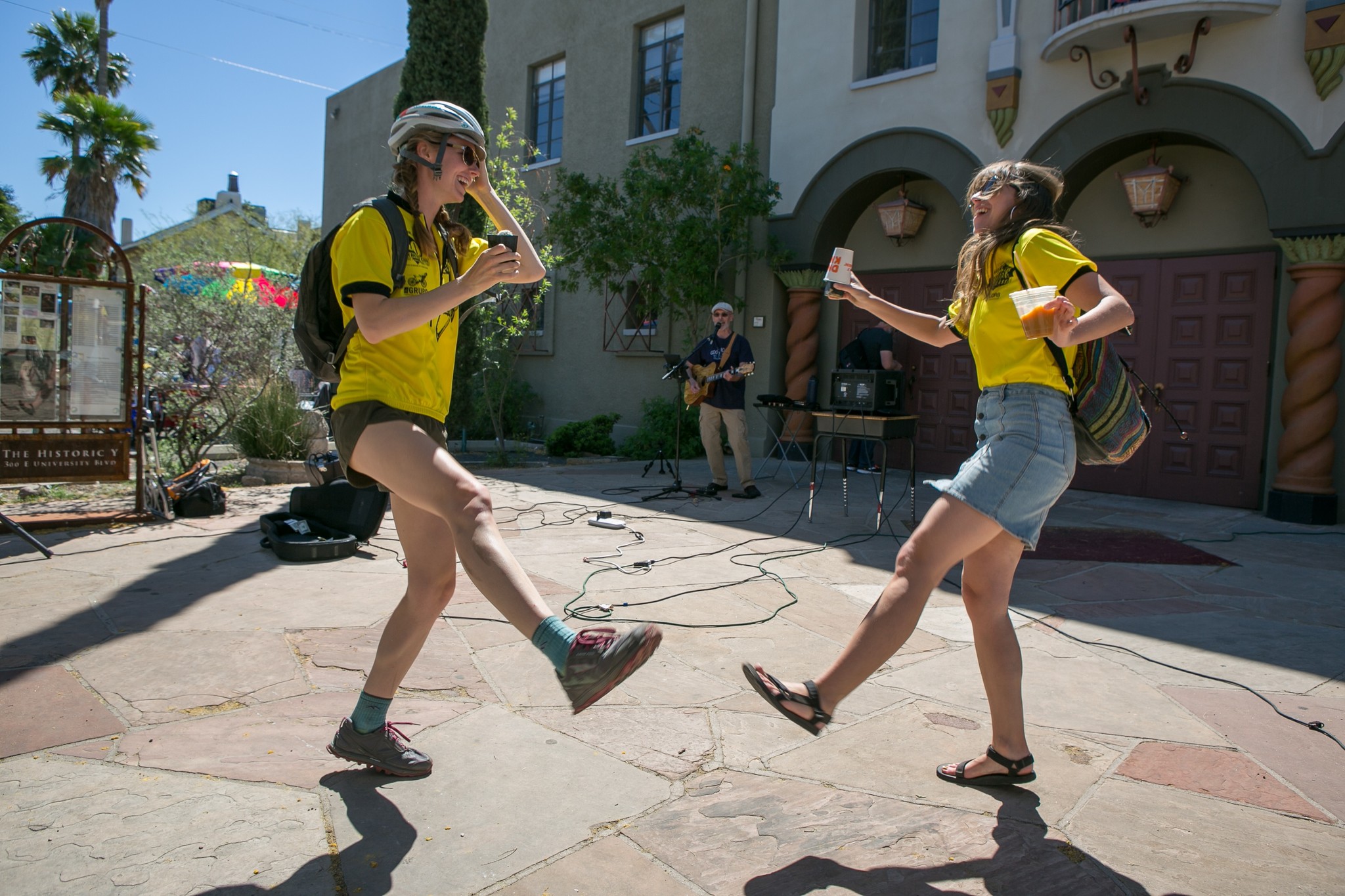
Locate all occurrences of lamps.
[875,173,930,248]
[1115,143,1193,230]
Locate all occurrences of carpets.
[1021,526,1241,566]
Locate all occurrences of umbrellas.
[154,261,301,309]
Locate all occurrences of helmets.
[388,101,486,154]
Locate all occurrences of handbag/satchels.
[158,461,227,517]
[1067,332,1154,467]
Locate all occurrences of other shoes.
[857,466,886,474]
[706,482,728,491]
[744,485,761,496]
[847,463,857,471]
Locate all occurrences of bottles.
[806,375,818,405]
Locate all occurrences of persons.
[15,359,48,409]
[848,320,904,473]
[742,162,1135,787]
[132,323,234,429]
[687,302,761,499]
[326,101,664,779]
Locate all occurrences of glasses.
[967,175,1021,208]
[713,312,727,317]
[426,139,481,169]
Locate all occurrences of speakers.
[830,369,906,415]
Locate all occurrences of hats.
[712,302,734,314]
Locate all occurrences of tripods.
[641,334,723,501]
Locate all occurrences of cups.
[1009,285,1058,340]
[823,248,854,286]
[487,234,518,264]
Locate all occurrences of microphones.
[710,322,721,344]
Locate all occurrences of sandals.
[936,745,1036,786]
[741,662,829,736]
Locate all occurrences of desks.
[752,402,820,489]
[807,410,919,532]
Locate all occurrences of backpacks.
[838,328,870,370]
[291,198,458,383]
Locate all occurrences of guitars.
[683,360,756,407]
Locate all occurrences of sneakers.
[325,717,432,776]
[556,623,661,715]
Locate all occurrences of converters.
[600,510,612,518]
